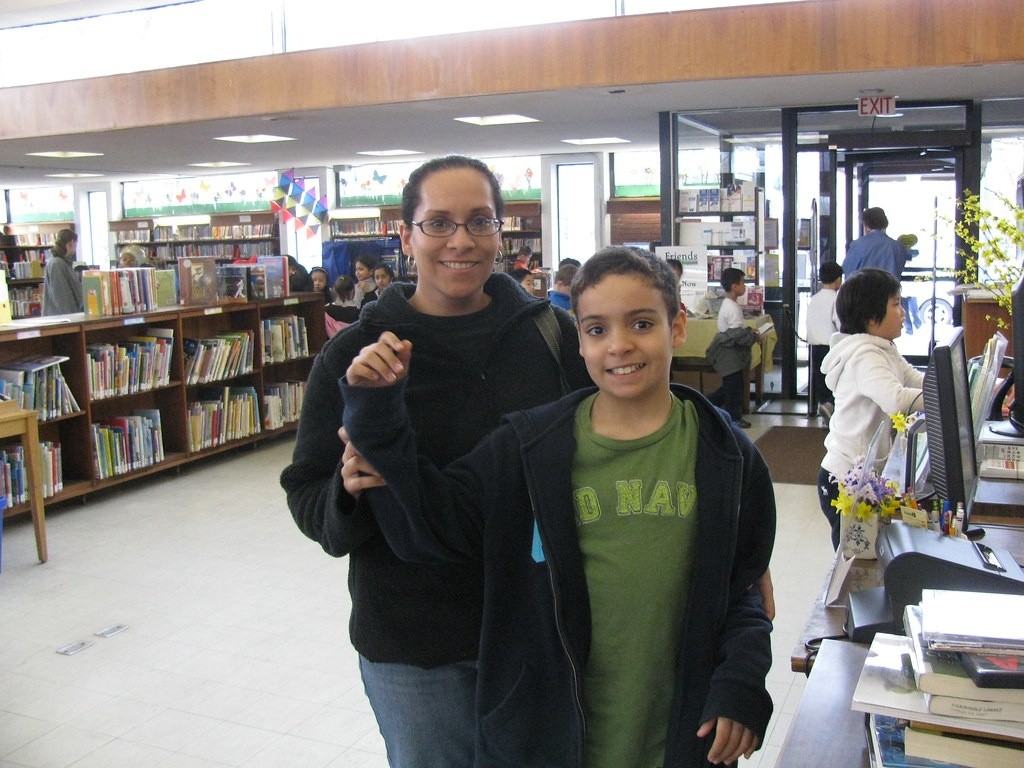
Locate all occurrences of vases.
[837,506,885,560]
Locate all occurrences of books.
[0,233,58,317]
[494,216,541,274]
[851,588,1024,768]
[81,255,289,315]
[118,224,274,259]
[0,315,309,507]
[331,219,402,241]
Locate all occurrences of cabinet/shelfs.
[109,209,279,288]
[0,224,76,319]
[677,185,760,290]
[327,198,544,297]
[0,293,330,519]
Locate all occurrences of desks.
[961,288,1015,379]
[771,478,1022,767]
[0,399,49,562]
[673,314,776,416]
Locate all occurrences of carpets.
[752,426,830,488]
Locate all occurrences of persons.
[817,269,1016,552]
[279,154,775,768]
[707,268,767,428]
[900,281,922,337]
[310,267,328,291]
[41,228,84,316]
[843,206,907,282]
[806,262,843,425]
[321,271,361,340]
[514,246,532,270]
[549,258,582,318]
[353,255,375,307]
[283,255,314,292]
[119,253,136,268]
[359,264,395,312]
[338,246,774,768]
[510,268,535,294]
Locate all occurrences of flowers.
[955,187,1024,329]
[832,461,901,526]
[889,413,912,434]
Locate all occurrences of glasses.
[410,214,504,238]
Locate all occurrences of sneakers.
[731,418,751,428]
[819,402,834,426]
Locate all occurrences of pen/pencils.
[939,499,964,538]
[917,504,922,511]
[904,498,910,507]
[908,487,918,510]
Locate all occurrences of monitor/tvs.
[904,326,980,533]
